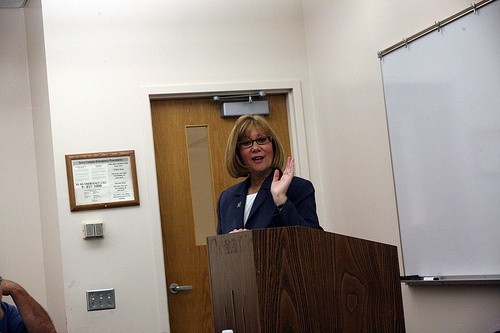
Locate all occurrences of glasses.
[237,134,272,149]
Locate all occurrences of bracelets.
[275,203,285,210]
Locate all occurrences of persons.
[216,114,324,235]
[0,276,56,333]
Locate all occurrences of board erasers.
[401,275,420,280]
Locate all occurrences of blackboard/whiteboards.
[379,0,500,287]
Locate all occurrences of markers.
[423,277,440,280]
